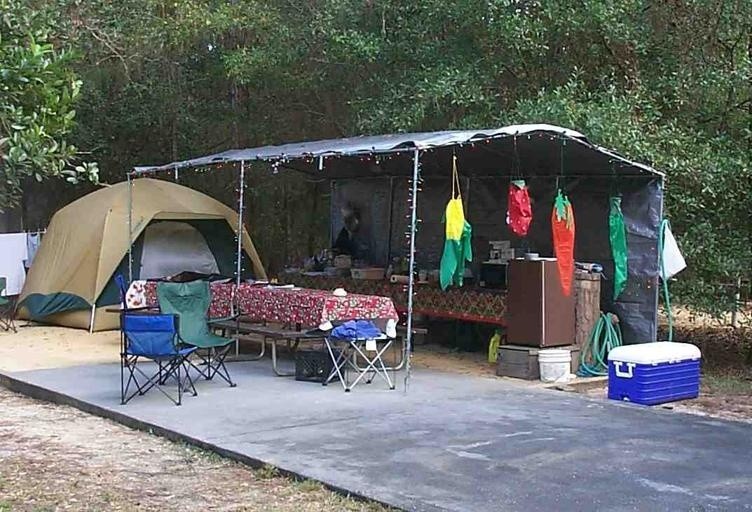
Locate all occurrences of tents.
[9,175,267,336]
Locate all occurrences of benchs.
[213,313,429,376]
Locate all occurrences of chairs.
[121,280,236,406]
[0,277,19,332]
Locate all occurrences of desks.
[277,268,506,350]
[146,276,407,375]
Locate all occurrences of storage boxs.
[606,340,701,405]
